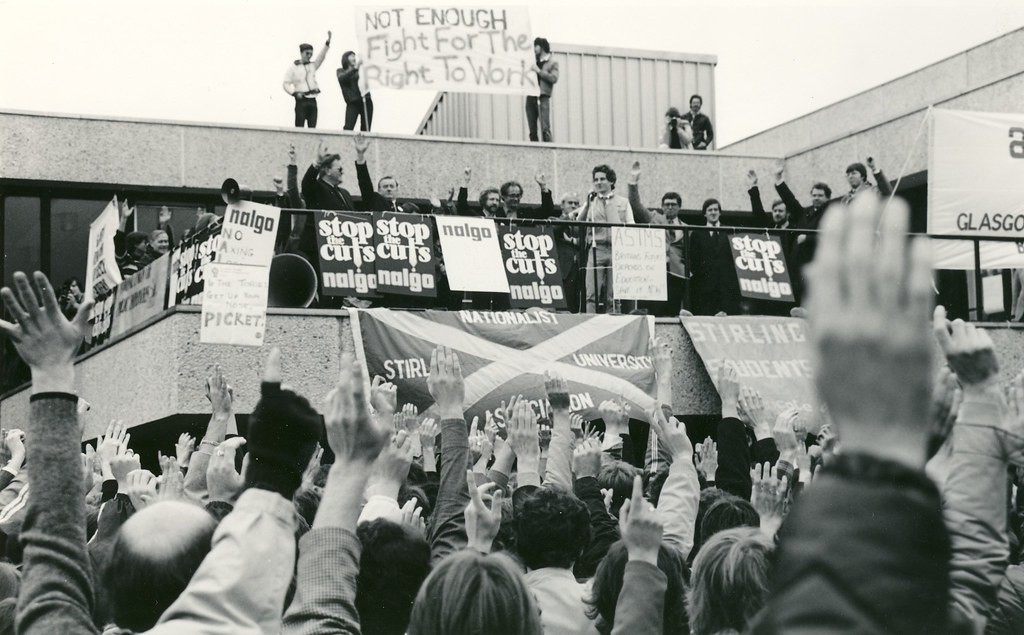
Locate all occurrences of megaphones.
[221,178,254,204]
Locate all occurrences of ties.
[774,224,782,237]
[711,224,719,245]
[668,219,676,242]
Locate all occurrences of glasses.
[305,52,314,56]
[507,193,526,199]
[339,166,343,173]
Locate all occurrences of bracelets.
[541,188,548,190]
[202,439,220,446]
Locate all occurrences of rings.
[794,429,798,432]
[217,450,224,457]
[390,435,401,448]
[648,507,655,512]
[491,424,494,427]
[476,442,481,447]
[125,450,132,455]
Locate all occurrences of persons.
[55,276,85,321]
[283,31,332,128]
[493,173,557,227]
[0,133,1024,635]
[627,162,693,317]
[525,37,559,142]
[548,191,588,314]
[679,95,714,150]
[658,107,694,150]
[336,51,373,131]
[575,164,636,314]
